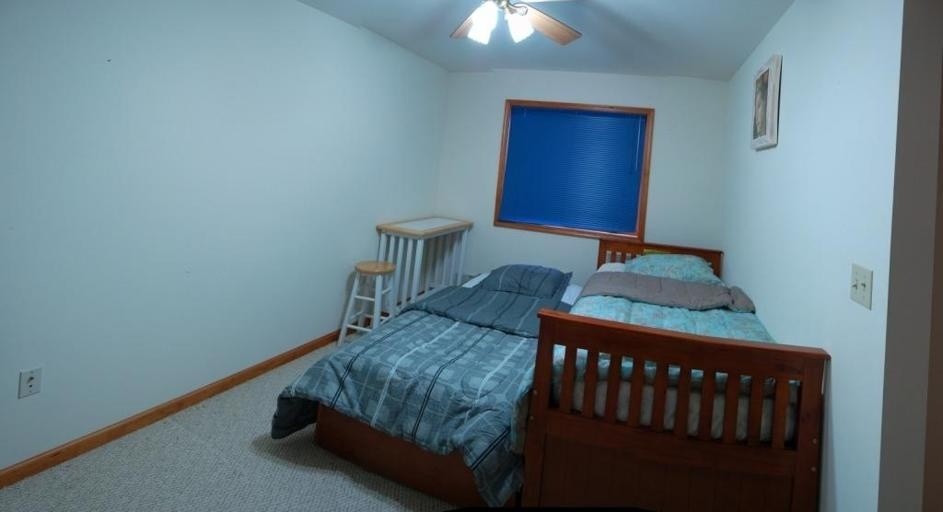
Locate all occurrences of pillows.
[480,263,564,300]
[623,252,722,284]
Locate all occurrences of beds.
[314,265,584,511]
[520,239,832,511]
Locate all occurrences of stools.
[335,260,394,346]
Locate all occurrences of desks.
[376,213,475,319]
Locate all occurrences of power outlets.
[19,368,40,398]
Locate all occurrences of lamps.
[449,1,584,46]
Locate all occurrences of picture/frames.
[751,55,781,152]
